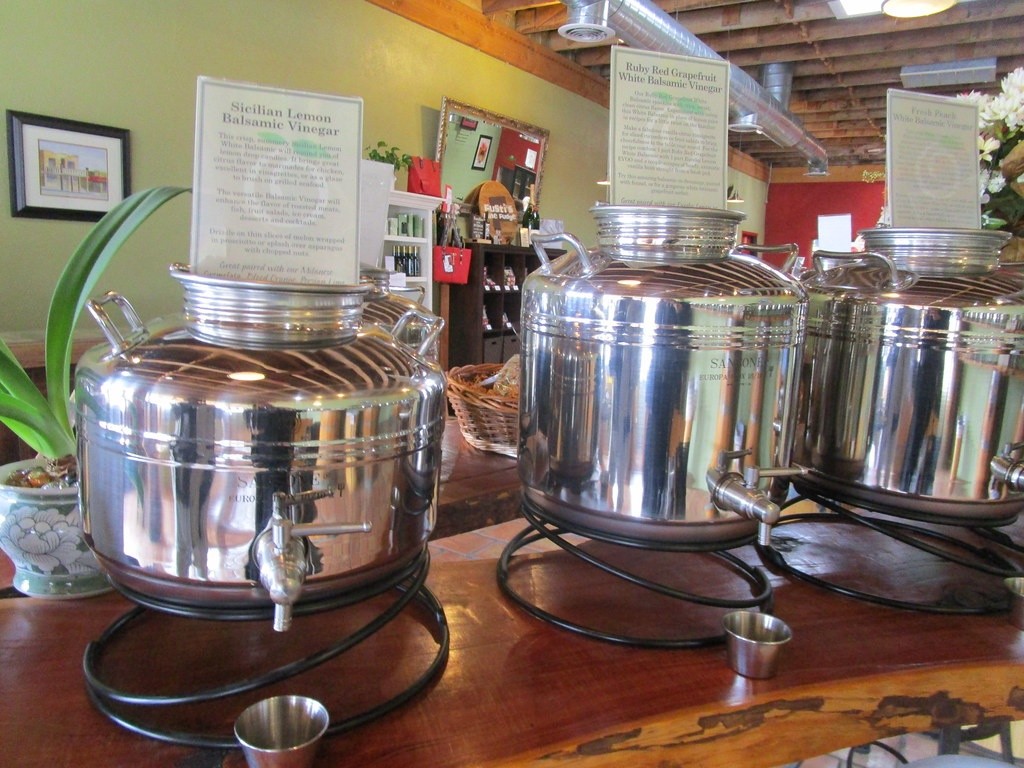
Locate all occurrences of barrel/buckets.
[786,227,1024,519]
[517,201,809,550]
[68,261,449,631]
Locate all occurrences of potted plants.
[0,183,195,605]
[364,141,415,185]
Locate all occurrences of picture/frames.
[461,116,479,131]
[6,108,131,223]
[471,135,493,171]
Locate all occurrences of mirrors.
[436,93,552,219]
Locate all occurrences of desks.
[0,502,1023,768]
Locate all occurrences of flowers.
[946,64,1024,239]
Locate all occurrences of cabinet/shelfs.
[447,244,568,367]
[378,186,447,349]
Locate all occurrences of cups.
[721,611,793,680]
[384,255,394,272]
[233,695,330,768]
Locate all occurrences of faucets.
[267,486,350,532]
[258,517,375,634]
[704,447,755,499]
[986,438,1024,498]
[715,465,810,549]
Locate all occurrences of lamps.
[828,0,962,22]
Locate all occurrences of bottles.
[482,211,490,240]
[392,245,420,277]
[522,207,540,231]
